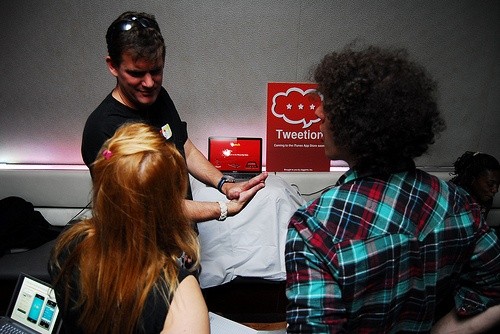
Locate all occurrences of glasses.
[112,16,158,31]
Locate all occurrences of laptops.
[0,271,64,334]
[206,136,263,187]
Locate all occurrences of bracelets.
[216,199,228,223]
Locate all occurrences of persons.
[283,38,499,333]
[446,151,499,220]
[80,12,268,234]
[48,121,211,334]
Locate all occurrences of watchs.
[216,174,236,191]
